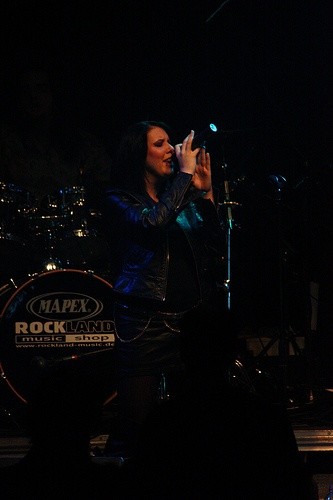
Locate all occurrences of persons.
[0,354,318,500]
[99,120,228,455]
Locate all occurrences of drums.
[0,219,25,241]
[33,226,74,260]
[60,185,88,209]
[0,182,22,206]
[1,268,117,414]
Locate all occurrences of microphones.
[172,123,218,162]
